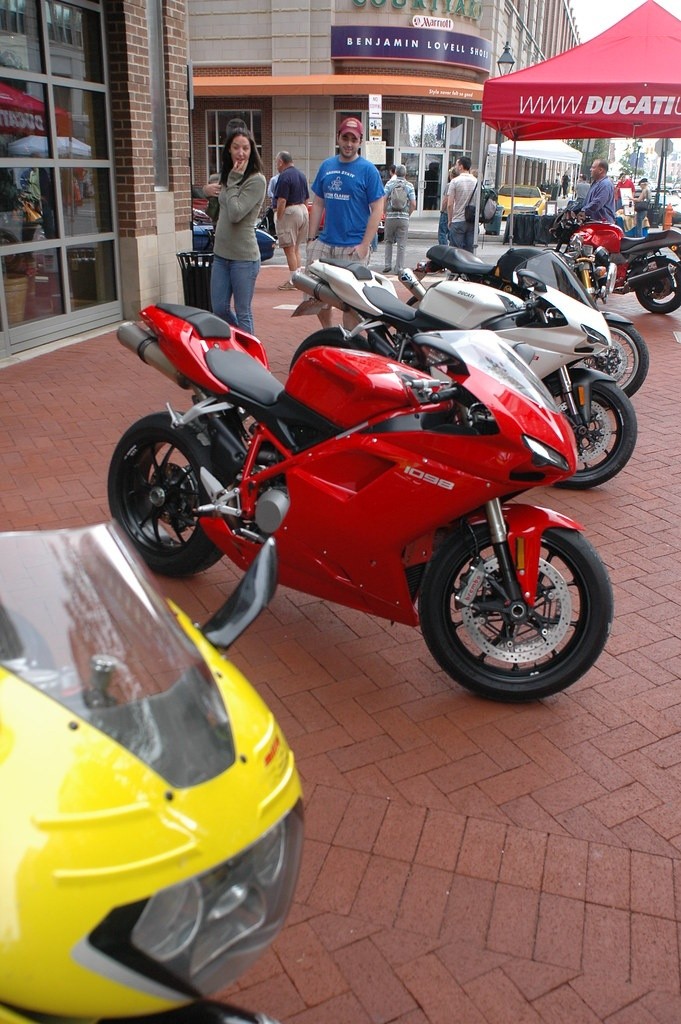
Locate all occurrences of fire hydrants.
[663,203,674,231]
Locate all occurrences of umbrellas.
[8,137,91,156]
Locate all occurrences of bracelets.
[305,237,315,242]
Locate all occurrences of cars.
[193,220,276,262]
[613,181,618,188]
[498,184,552,220]
[192,207,213,225]
[0,180,26,269]
[307,202,386,243]
[633,182,681,206]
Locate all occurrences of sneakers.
[278,280,299,291]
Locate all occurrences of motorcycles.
[398,200,650,412]
[543,196,681,314]
[106,303,615,705]
[289,250,639,491]
[0,517,306,1024]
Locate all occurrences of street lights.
[495,41,515,194]
[634,138,643,183]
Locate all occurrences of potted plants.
[0,169,30,328]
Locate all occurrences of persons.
[74,167,88,200]
[303,117,385,335]
[388,165,397,181]
[612,174,651,225]
[577,159,615,225]
[437,155,482,255]
[201,127,268,335]
[378,165,391,185]
[555,171,592,205]
[19,151,57,244]
[381,165,416,275]
[268,151,310,292]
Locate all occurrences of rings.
[215,191,218,195]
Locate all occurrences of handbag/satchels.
[205,196,219,220]
[465,205,476,222]
[635,202,649,212]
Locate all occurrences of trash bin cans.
[67,247,98,301]
[484,205,505,235]
[175,250,213,314]
[649,202,662,228]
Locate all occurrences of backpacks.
[480,184,499,222]
[390,179,409,210]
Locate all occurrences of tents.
[484,138,583,199]
[0,82,75,240]
[471,0,681,261]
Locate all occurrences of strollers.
[255,202,278,241]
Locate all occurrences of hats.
[638,178,647,185]
[338,118,364,138]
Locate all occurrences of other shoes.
[382,267,392,272]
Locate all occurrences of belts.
[286,202,304,207]
